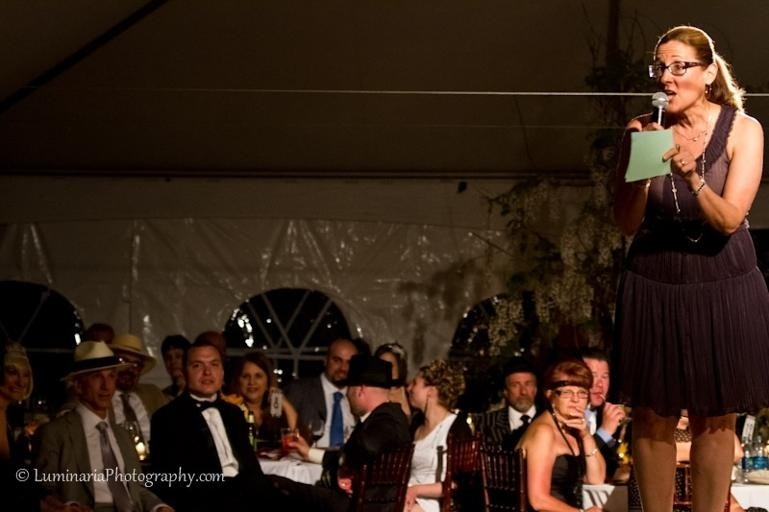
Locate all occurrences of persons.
[605,26,769,512]
[1,323,769,512]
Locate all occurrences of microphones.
[650,92,667,132]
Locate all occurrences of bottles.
[743,435,769,469]
[246,410,257,450]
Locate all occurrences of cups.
[123,421,138,441]
[279,427,301,453]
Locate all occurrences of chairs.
[348,419,732,512]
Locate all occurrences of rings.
[680,160,685,165]
[675,144,682,152]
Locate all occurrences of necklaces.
[668,103,718,242]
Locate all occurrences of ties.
[520,414,530,426]
[119,393,146,462]
[95,421,132,512]
[330,391,344,446]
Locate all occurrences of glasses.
[554,388,589,399]
[648,61,705,78]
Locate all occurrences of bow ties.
[200,400,218,411]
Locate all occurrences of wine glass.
[309,417,326,448]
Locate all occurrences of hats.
[336,354,401,388]
[60,334,156,380]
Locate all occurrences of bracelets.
[689,180,707,196]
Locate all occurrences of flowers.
[425,126,631,369]
